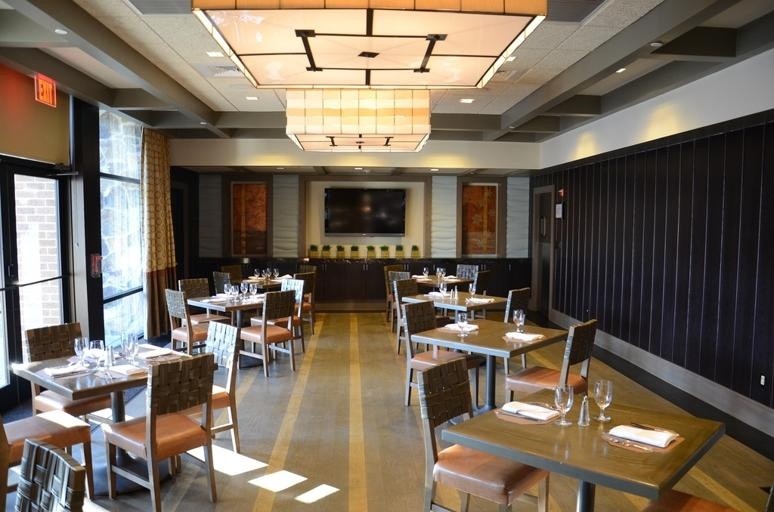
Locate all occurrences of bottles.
[450,285,459,299]
[309,244,421,258]
[577,395,591,427]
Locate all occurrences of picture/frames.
[461,182,500,259]
[230,180,273,258]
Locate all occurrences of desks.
[409,318,568,425]
[188,295,273,367]
[12,341,196,495]
[441,387,727,508]
[411,272,474,288]
[401,292,509,320]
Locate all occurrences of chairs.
[402,301,469,406]
[14,439,87,511]
[240,291,296,377]
[415,359,549,512]
[644,485,772,511]
[249,278,305,354]
[1,403,94,502]
[196,321,241,452]
[506,320,598,409]
[103,353,217,511]
[25,325,122,435]
[179,270,314,334]
[164,288,210,355]
[384,264,531,358]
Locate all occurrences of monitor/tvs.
[323,187,408,239]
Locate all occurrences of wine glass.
[512,309,524,335]
[254,266,279,282]
[468,283,476,300]
[73,329,140,373]
[422,267,446,282]
[223,282,257,303]
[593,379,613,422]
[439,282,447,300]
[554,384,574,426]
[456,313,467,337]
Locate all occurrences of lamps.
[285,88,431,153]
